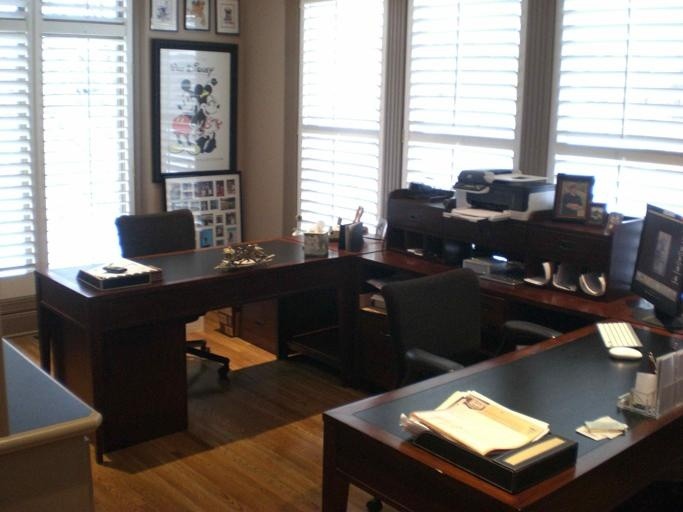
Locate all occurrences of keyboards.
[596,320,642,350]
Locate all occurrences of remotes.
[103,262,127,274]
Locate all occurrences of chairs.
[378,266,564,391]
[113,207,232,380]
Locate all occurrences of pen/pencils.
[358,209,364,223]
[354,206,361,224]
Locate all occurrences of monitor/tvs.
[628,204,683,331]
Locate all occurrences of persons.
[562,185,584,216]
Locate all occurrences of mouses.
[607,345,642,363]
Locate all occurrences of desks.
[32,236,344,466]
[320,320,682,510]
[0,335,107,512]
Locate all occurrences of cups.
[632,372,658,409]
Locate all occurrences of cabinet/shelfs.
[350,250,683,400]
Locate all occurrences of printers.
[443,168,557,226]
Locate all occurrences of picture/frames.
[147,0,179,32]
[161,170,245,252]
[213,0,242,36]
[148,36,240,184]
[182,0,211,31]
[549,172,597,226]
